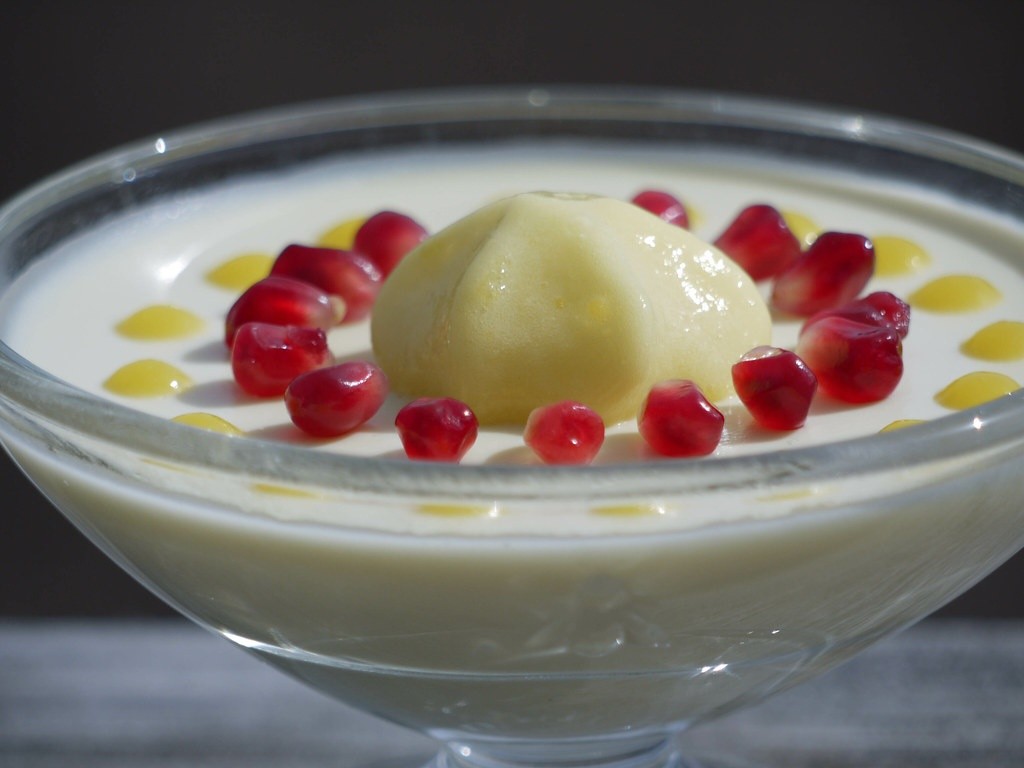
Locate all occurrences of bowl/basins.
[0,88,1024,768]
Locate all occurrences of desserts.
[0,159,1023,734]
